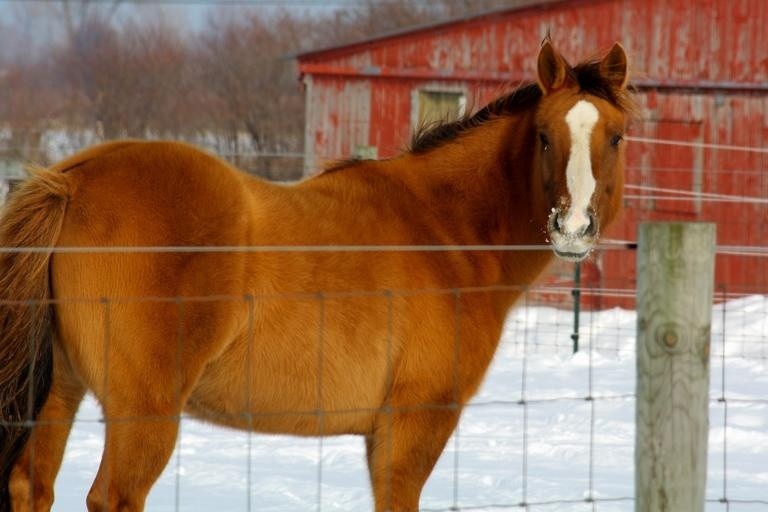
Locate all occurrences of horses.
[0,23,655,512]
